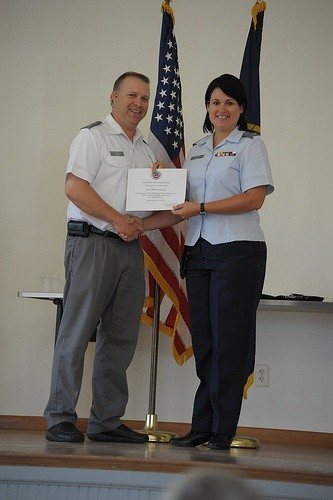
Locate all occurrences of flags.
[240,0,266,399]
[141,0,193,365]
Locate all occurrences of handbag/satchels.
[67,221,90,237]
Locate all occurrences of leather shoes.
[87,423,149,443]
[45,422,84,442]
[207,434,234,450]
[170,431,212,447]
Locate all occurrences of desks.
[17,290,333,349]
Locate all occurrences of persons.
[44,72,176,444]
[117,74,274,451]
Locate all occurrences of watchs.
[200,203,206,215]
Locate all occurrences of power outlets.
[255,364,269,387]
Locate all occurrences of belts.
[88,225,120,239]
